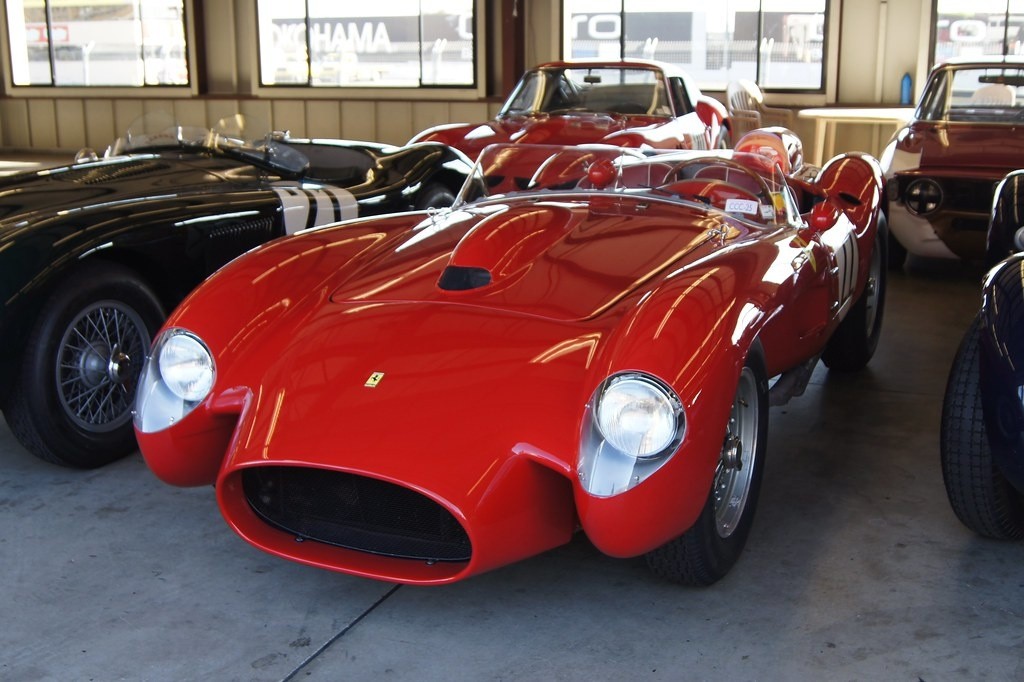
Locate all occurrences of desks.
[798,107,915,166]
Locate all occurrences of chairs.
[576,163,676,191]
[973,83,1014,107]
[694,165,798,217]
[726,80,793,148]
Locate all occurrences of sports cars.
[405,57,733,197]
[0,124,486,470]
[127,122,892,589]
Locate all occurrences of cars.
[939,166,1024,544]
[872,55,1024,275]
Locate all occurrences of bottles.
[901,73,911,104]
